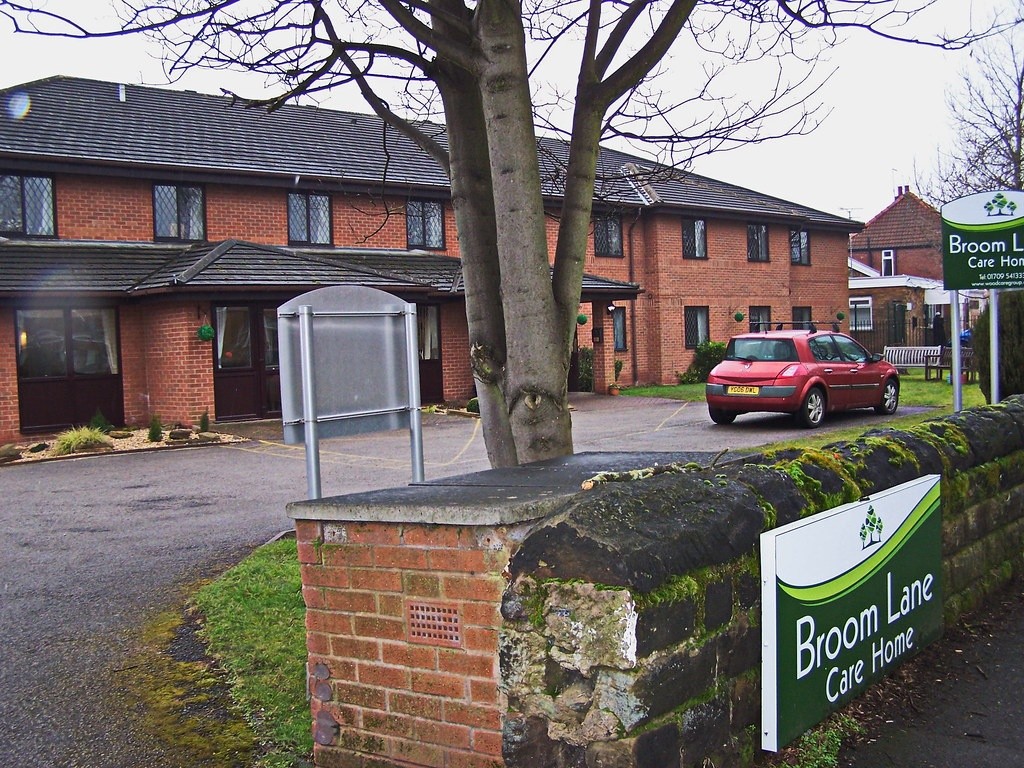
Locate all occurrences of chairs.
[814,345,830,361]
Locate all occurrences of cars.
[704,319,900,428]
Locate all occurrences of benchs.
[883,345,942,379]
[923,346,979,383]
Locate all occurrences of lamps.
[606,304,615,314]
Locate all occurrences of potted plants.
[609,383,620,395]
